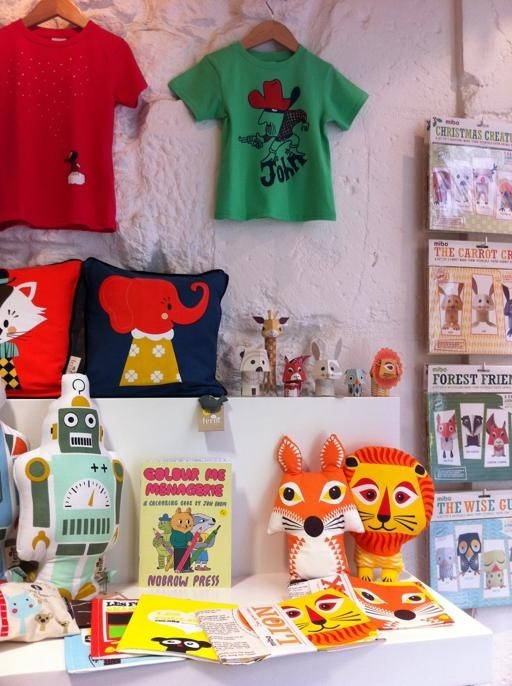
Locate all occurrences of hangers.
[20,0,89,29]
[240,0,299,57]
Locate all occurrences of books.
[66,626,187,677]
[119,593,240,665]
[138,459,235,593]
[347,574,455,630]
[279,586,381,651]
[241,605,318,661]
[64,599,91,631]
[289,573,362,612]
[91,597,145,661]
[199,610,268,664]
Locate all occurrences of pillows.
[0,255,232,399]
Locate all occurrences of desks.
[0,574,495,686]
